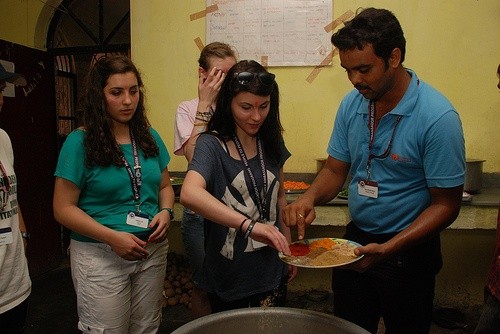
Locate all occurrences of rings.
[296,214,305,219]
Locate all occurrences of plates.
[336,192,348,198]
[278,238,364,268]
[285,189,309,193]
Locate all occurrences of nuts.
[162,251,193,310]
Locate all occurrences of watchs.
[160,208,174,220]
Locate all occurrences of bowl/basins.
[170,178,184,196]
[169,308,371,334]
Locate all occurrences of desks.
[167,188,500,308]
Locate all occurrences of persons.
[282,8,467,334]
[173,41,238,320]
[179,60,299,313]
[0,62,32,334]
[53,55,174,334]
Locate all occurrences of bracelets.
[240,218,250,232]
[21,231,30,239]
[244,220,256,239]
[194,110,212,126]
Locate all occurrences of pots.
[464,159,486,192]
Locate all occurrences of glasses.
[231,72,275,86]
